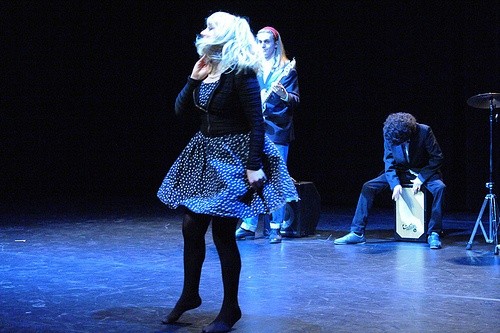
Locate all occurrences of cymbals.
[467,92,500,109]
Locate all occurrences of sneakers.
[334,231,366,245]
[426,232,442,249]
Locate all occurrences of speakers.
[263,181,322,237]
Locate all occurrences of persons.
[157,11,299,333]
[235,26,300,243]
[333,111,446,249]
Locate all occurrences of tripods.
[466,98,500,256]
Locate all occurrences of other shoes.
[234,226,257,240]
[268,222,282,243]
